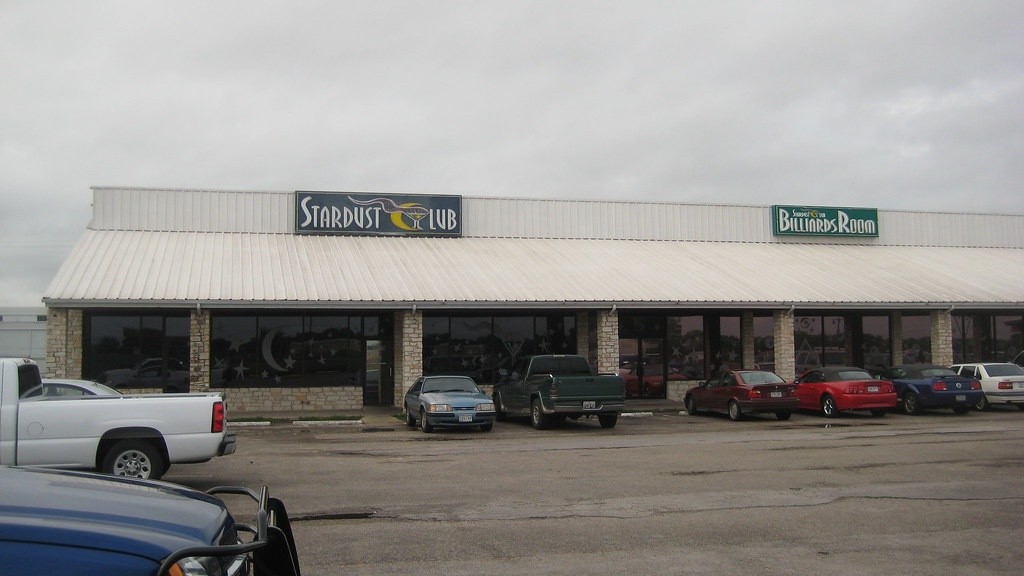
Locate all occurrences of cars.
[949,364,1024,411]
[404,376,495,434]
[1,465,304,576]
[620,362,686,399]
[786,364,897,418]
[684,368,801,421]
[873,364,983,416]
[41,376,124,396]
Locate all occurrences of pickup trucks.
[1,356,236,483]
[99,357,192,393]
[493,353,628,431]
